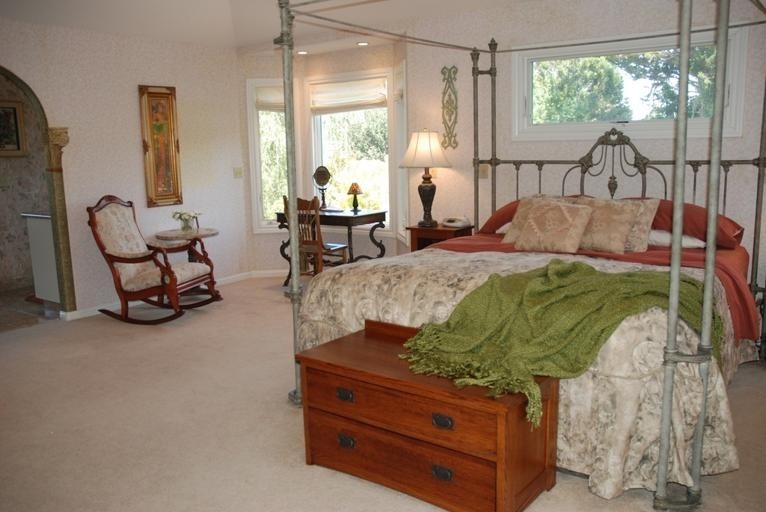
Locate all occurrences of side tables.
[155,226,223,307]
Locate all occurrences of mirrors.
[313,165,330,210]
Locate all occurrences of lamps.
[347,181,363,211]
[397,131,451,229]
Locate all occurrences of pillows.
[501,194,662,254]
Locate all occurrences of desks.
[274,208,387,287]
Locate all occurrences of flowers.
[170,207,204,220]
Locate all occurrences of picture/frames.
[0,100,30,160]
[139,84,184,209]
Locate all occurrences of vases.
[180,218,195,232]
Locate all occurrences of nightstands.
[405,221,474,253]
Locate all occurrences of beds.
[273,0,766,512]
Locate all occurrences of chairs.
[283,194,349,277]
[85,196,223,325]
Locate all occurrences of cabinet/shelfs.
[294,320,560,512]
